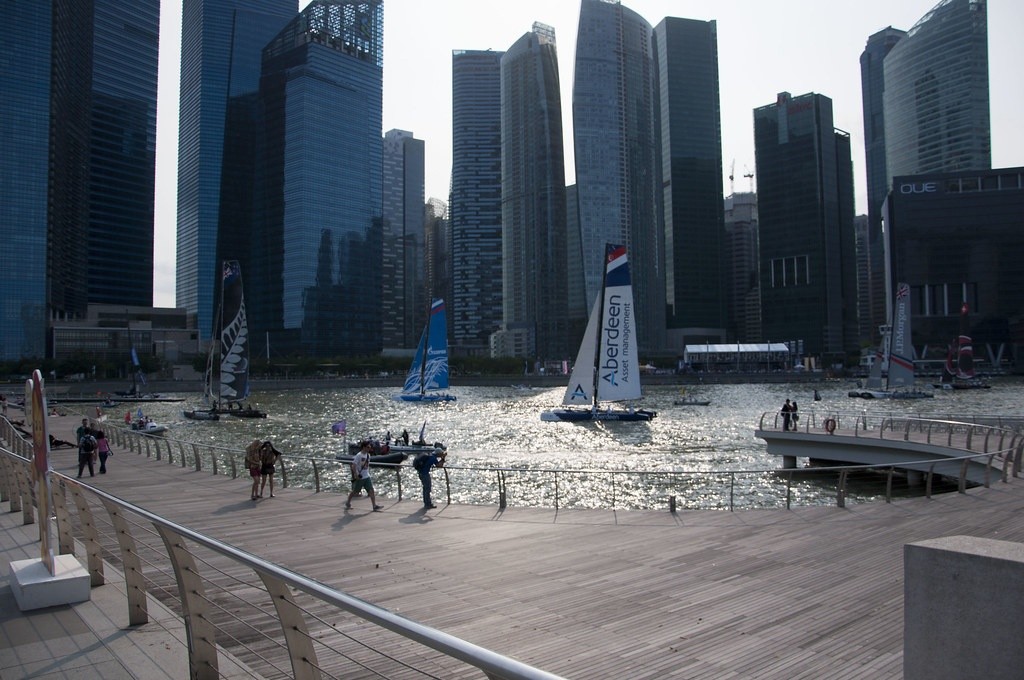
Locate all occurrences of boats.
[674,393,712,406]
[336,438,448,466]
[512,382,549,398]
[126,422,171,434]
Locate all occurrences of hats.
[432,447,443,455]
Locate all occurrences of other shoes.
[255,494,263,498]
[373,505,384,511]
[345,501,353,510]
[425,504,437,510]
[270,494,275,497]
[251,495,257,500]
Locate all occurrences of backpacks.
[82,435,94,452]
[413,451,437,471]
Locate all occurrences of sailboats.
[934,303,992,389]
[113,347,160,398]
[389,297,458,402]
[183,260,268,421]
[848,281,933,399]
[540,242,658,421]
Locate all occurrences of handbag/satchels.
[350,468,357,483]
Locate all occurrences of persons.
[781,399,797,431]
[370,429,426,456]
[345,441,384,511]
[1,398,7,414]
[76,422,114,478]
[418,448,446,508]
[138,416,152,430]
[246,440,282,500]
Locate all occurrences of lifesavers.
[826,419,836,432]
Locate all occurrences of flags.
[332,420,345,434]
